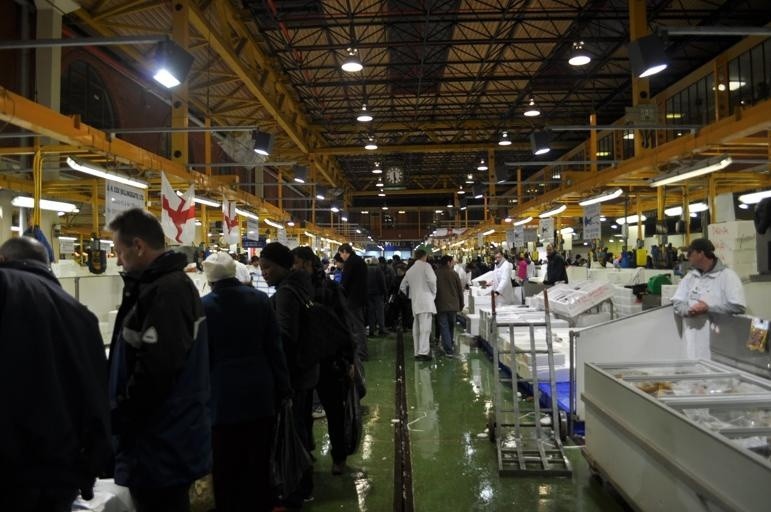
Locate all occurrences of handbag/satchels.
[273,285,350,376]
[264,400,313,508]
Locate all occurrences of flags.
[160,170,195,245]
[221,196,240,243]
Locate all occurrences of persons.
[199,244,393,511]
[452,245,568,305]
[573,244,678,270]
[0,235,115,512]
[392,249,465,361]
[670,239,746,317]
[109,209,214,512]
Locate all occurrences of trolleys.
[484,287,575,483]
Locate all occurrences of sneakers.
[312,404,326,419]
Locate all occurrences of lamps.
[236,167,385,251]
[66,153,152,191]
[253,129,275,160]
[577,185,626,208]
[648,150,734,190]
[151,34,196,91]
[340,38,595,156]
[539,201,568,220]
[175,187,223,208]
[625,30,670,81]
[435,162,534,239]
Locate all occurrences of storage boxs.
[705,218,760,281]
[468,275,677,381]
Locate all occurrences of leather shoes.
[416,338,455,361]
[331,460,345,474]
[367,326,408,338]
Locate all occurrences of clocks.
[386,167,404,185]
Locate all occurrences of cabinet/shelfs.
[579,309,771,510]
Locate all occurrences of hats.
[200,251,235,282]
[681,239,715,251]
